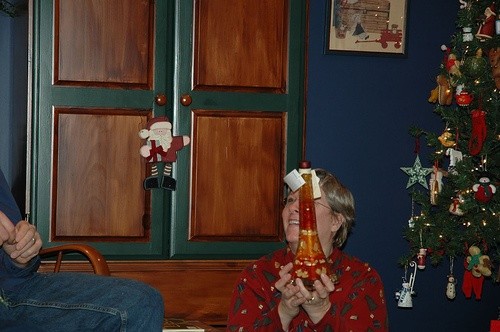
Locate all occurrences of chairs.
[38,244,113,277]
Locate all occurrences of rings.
[307,297,314,302]
[32,238,35,241]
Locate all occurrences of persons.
[0,169,164,332]
[475,2,495,42]
[226,168,387,332]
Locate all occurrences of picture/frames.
[324,0,411,59]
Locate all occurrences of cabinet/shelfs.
[24,0,309,327]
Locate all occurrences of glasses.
[284,196,334,212]
